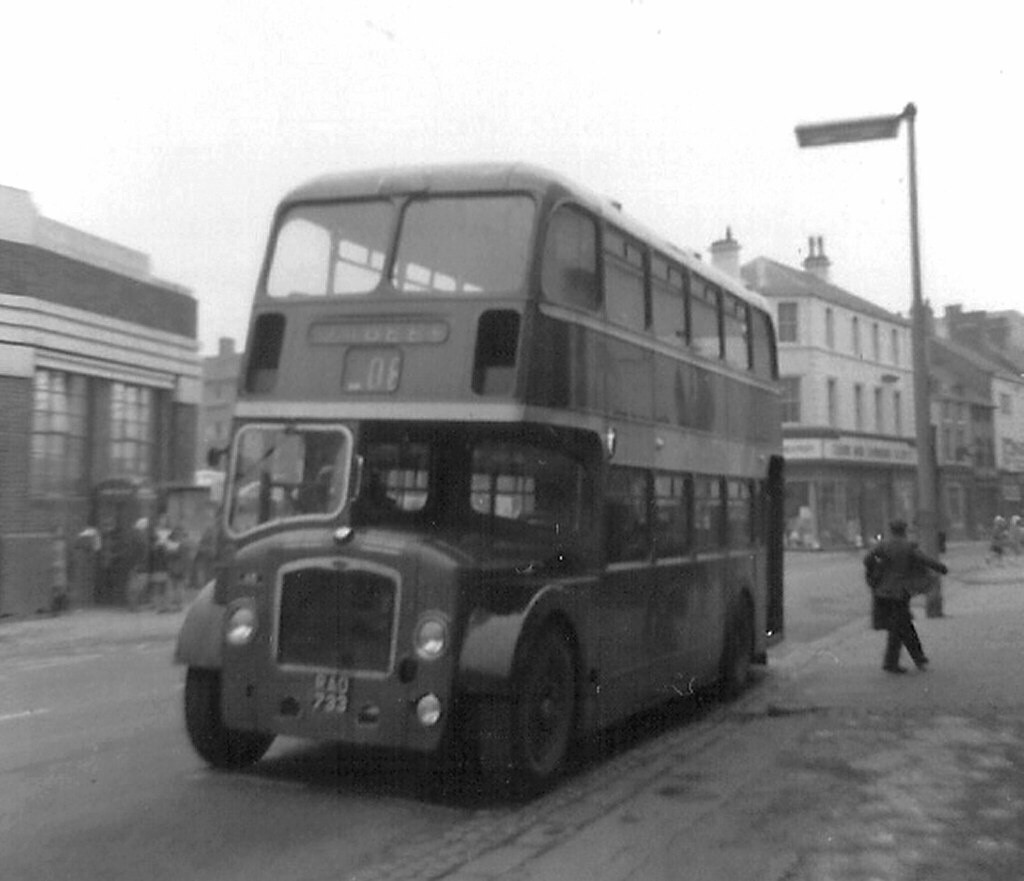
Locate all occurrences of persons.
[43,511,220,614]
[864,520,950,672]
[983,515,1024,563]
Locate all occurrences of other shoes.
[915,658,930,669]
[881,659,909,675]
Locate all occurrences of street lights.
[792,103,944,619]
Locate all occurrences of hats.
[890,514,907,530]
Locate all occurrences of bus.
[170,162,790,805]
